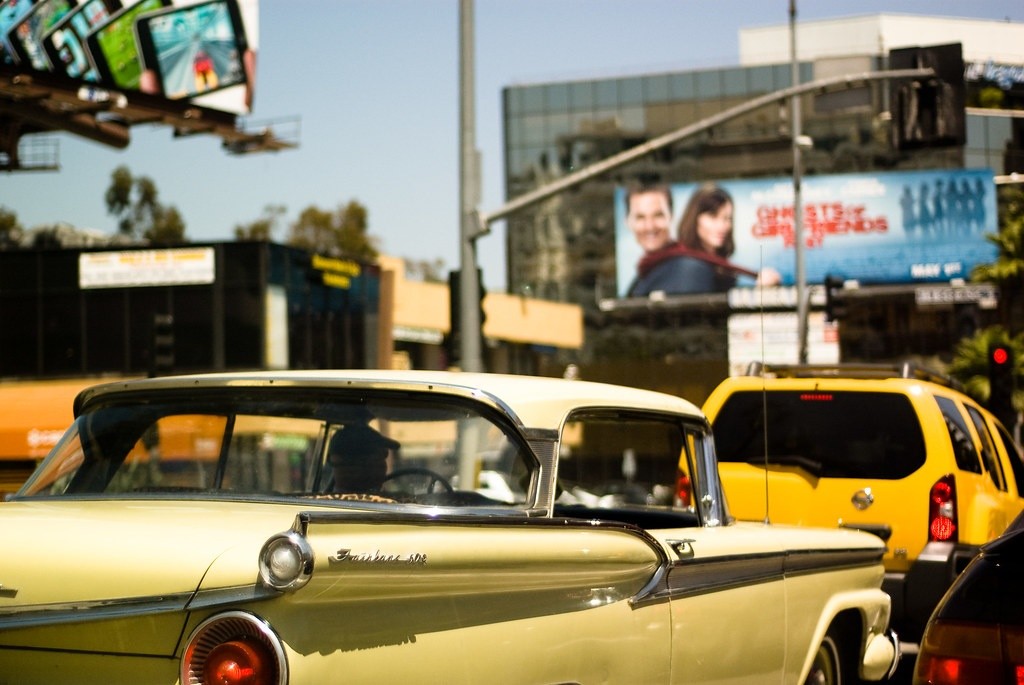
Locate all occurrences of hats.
[327,425,400,464]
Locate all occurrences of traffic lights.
[987,341,1016,427]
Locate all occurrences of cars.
[674,364,1024,685]
[0,371,912,685]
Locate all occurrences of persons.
[676,186,781,293]
[624,186,757,301]
[325,425,401,496]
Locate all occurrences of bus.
[0,234,397,500]
